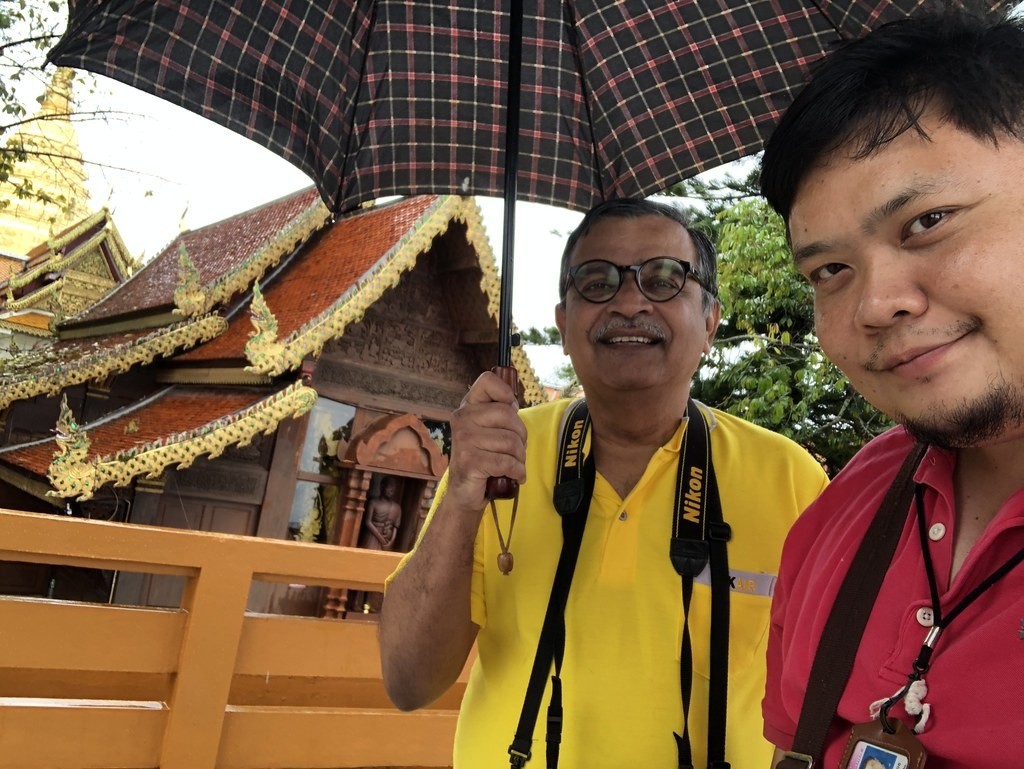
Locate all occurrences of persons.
[378,203,833,769]
[353,476,401,613]
[756,9,1023,769]
[860,757,887,769]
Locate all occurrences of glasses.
[562,255,719,304]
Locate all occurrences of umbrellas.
[41,0,1024,500]
